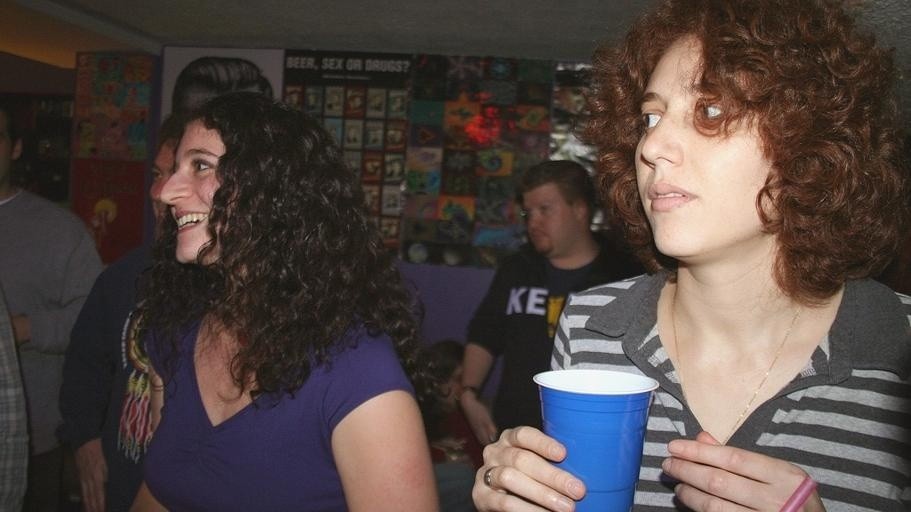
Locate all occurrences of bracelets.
[461,385,480,398]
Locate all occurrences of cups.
[530,367,658,511]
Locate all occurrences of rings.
[483,466,498,488]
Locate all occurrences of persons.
[1,92,437,512]
[458,162,647,449]
[472,0,911,511]
[172,57,272,113]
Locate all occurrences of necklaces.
[671,282,801,445]
